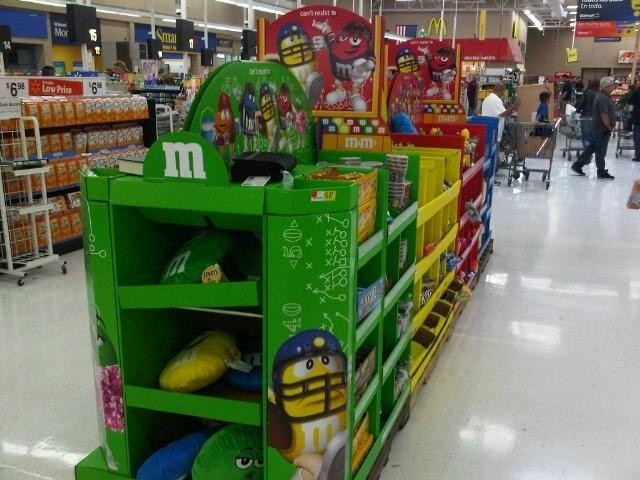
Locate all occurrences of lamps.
[16,0,415,44]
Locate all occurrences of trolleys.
[0,115,70,286]
[494,115,527,178]
[610,100,625,137]
[614,105,639,160]
[559,109,595,162]
[508,118,563,189]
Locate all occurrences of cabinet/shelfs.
[76,68,498,480]
[3,60,193,284]
[610,68,640,88]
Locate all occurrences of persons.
[529,92,553,137]
[560,74,640,179]
[113,61,129,80]
[481,82,520,186]
[42,66,55,76]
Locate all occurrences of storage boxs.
[516,83,555,157]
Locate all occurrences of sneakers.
[570,167,585,176]
[494,181,501,186]
[596,171,614,180]
